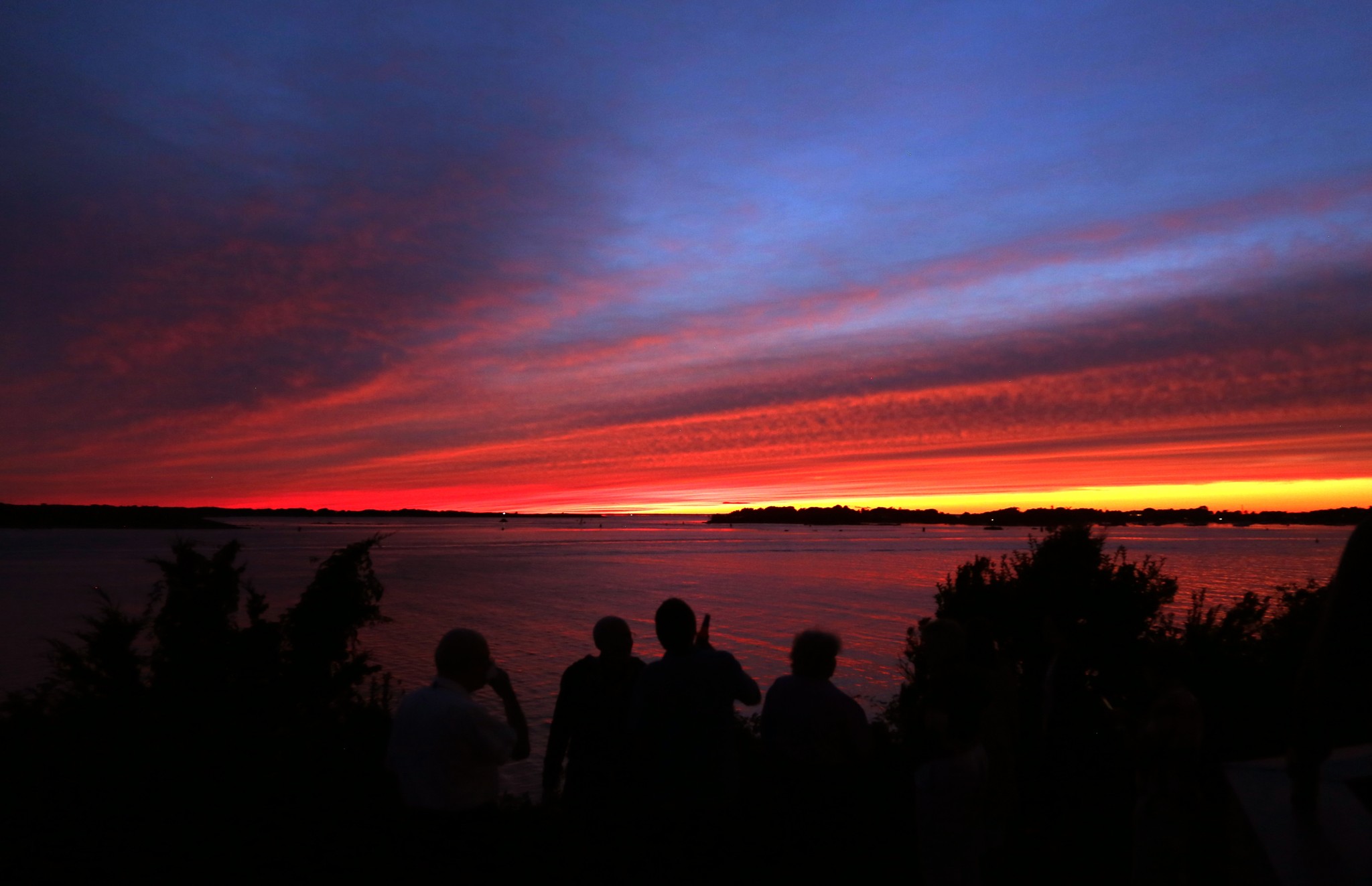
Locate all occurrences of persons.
[384,627,532,811]
[542,598,760,809]
[760,631,870,783]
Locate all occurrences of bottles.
[695,613,710,645]
